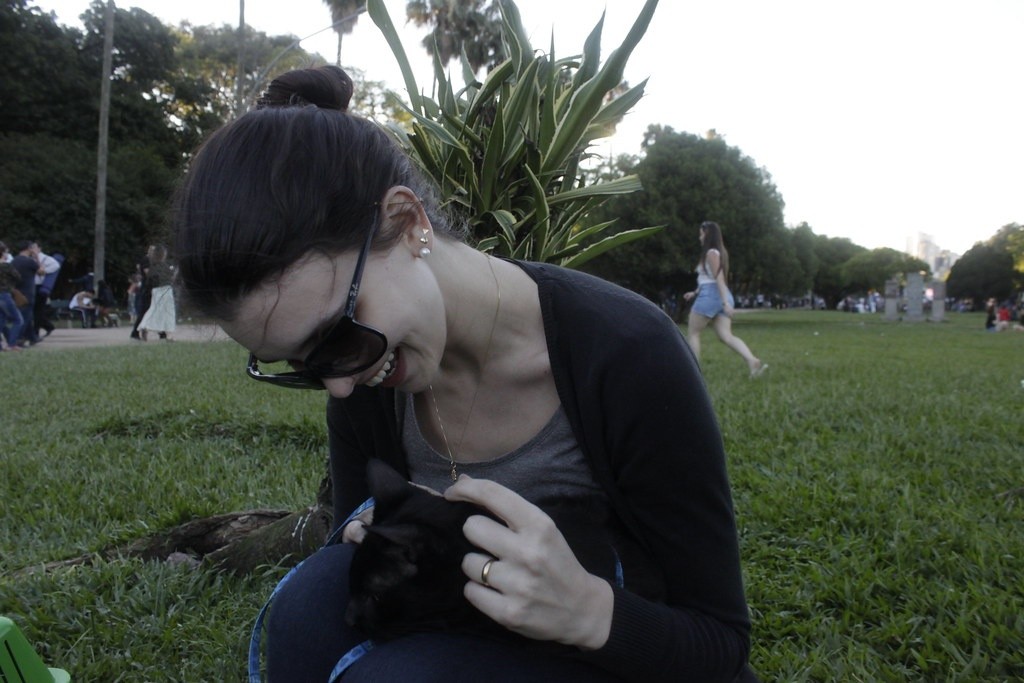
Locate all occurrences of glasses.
[701,221,708,229]
[245,201,388,391]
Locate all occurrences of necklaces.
[427,252,501,482]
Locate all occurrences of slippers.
[749,364,769,381]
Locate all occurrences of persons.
[837,289,878,314]
[0,240,61,351]
[127,243,179,340]
[985,298,1024,333]
[818,298,824,309]
[684,222,767,378]
[69,273,116,329]
[737,291,764,307]
[175,66,756,683]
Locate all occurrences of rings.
[482,558,496,586]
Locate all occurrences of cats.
[342,455,667,659]
